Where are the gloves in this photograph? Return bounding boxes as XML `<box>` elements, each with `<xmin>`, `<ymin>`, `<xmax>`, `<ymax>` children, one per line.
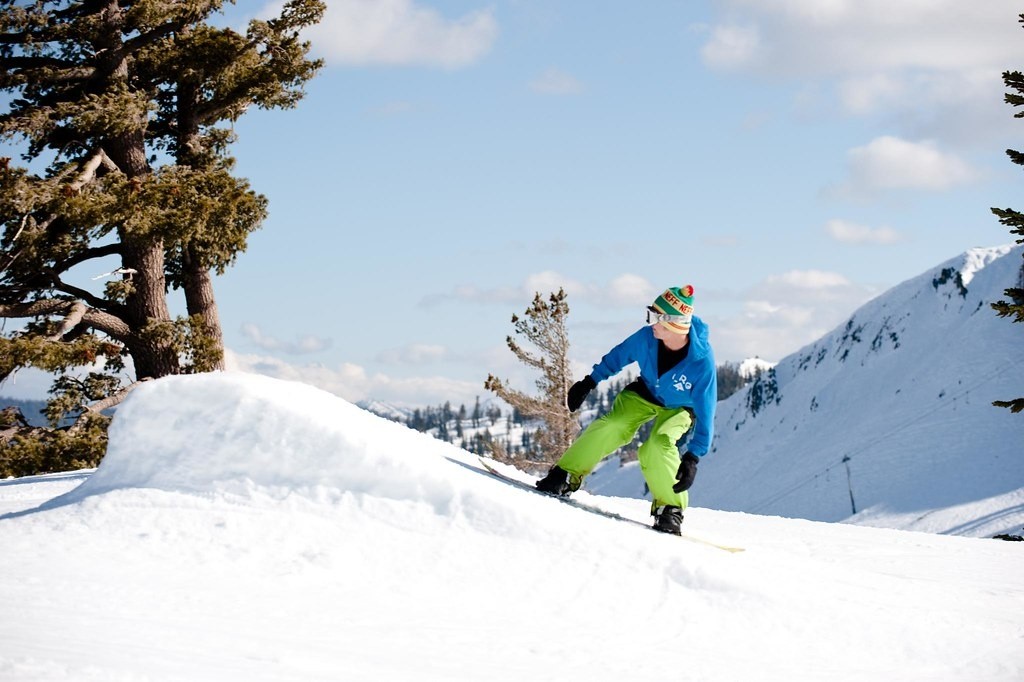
<box><xmin>568</xmin><ymin>375</ymin><xmax>596</xmax><ymax>412</ymax></box>
<box><xmin>672</xmin><ymin>452</ymin><xmax>699</xmax><ymax>493</ymax></box>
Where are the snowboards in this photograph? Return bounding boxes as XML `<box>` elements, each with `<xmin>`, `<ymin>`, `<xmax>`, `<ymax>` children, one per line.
<box><xmin>477</xmin><ymin>458</ymin><xmax>683</xmax><ymax>537</ymax></box>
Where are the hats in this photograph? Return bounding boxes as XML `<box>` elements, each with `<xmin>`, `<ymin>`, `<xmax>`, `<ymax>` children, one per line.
<box><xmin>653</xmin><ymin>285</ymin><xmax>695</xmax><ymax>334</ymax></box>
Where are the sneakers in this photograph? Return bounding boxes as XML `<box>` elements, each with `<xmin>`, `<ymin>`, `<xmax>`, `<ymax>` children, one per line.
<box><xmin>653</xmin><ymin>505</ymin><xmax>683</xmax><ymax>535</ymax></box>
<box><xmin>536</xmin><ymin>465</ymin><xmax>582</xmax><ymax>501</ymax></box>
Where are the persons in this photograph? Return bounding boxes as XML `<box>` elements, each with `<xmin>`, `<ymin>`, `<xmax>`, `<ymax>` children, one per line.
<box><xmin>535</xmin><ymin>285</ymin><xmax>718</xmax><ymax>535</ymax></box>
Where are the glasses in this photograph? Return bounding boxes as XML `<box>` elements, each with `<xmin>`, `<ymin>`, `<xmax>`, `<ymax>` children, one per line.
<box><xmin>645</xmin><ymin>306</ymin><xmax>692</xmax><ymax>326</ymax></box>
